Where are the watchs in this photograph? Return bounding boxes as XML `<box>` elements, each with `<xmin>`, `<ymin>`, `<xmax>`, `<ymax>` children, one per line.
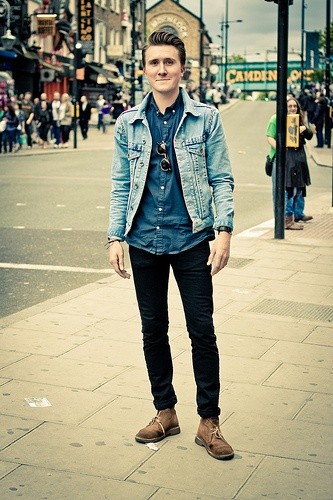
<box><xmin>211</xmin><ymin>222</ymin><xmax>233</xmax><ymax>239</ymax></box>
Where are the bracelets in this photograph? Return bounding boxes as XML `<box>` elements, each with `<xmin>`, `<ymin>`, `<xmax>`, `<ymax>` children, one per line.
<box><xmin>106</xmin><ymin>234</ymin><xmax>126</xmax><ymax>247</ymax></box>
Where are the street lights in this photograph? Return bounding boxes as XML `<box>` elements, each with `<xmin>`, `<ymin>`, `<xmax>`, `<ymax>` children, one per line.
<box><xmin>216</xmin><ymin>16</ymin><xmax>242</xmax><ymax>82</ymax></box>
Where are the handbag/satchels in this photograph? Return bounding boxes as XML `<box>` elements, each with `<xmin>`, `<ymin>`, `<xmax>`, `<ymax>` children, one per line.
<box><xmin>264</xmin><ymin>155</ymin><xmax>272</xmax><ymax>177</ymax></box>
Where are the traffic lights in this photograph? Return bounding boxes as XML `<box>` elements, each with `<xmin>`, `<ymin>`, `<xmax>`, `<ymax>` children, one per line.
<box><xmin>77</xmin><ymin>49</ymin><xmax>87</xmax><ymax>69</ymax></box>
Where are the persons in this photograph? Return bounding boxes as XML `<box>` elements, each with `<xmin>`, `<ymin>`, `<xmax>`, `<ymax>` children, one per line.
<box><xmin>295</xmin><ymin>104</ymin><xmax>313</xmax><ymax>222</ymax></box>
<box><xmin>1</xmin><ymin>86</ymin><xmax>333</xmax><ymax>152</ymax></box>
<box><xmin>105</xmin><ymin>32</ymin><xmax>234</xmax><ymax>460</ymax></box>
<box><xmin>264</xmin><ymin>99</ymin><xmax>304</xmax><ymax>234</ymax></box>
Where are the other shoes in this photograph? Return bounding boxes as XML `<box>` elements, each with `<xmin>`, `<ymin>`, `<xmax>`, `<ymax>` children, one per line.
<box><xmin>81</xmin><ymin>137</ymin><xmax>88</xmax><ymax>142</ymax></box>
<box><xmin>97</xmin><ymin>129</ymin><xmax>100</xmax><ymax>132</ymax></box>
<box><xmin>53</xmin><ymin>144</ymin><xmax>60</xmax><ymax>149</ymax></box>
<box><xmin>42</xmin><ymin>142</ymin><xmax>48</xmax><ymax>150</ymax></box>
<box><xmin>9</xmin><ymin>146</ymin><xmax>12</xmax><ymax>152</ymax></box>
<box><xmin>3</xmin><ymin>148</ymin><xmax>8</xmax><ymax>154</ymax></box>
<box><xmin>103</xmin><ymin>131</ymin><xmax>106</xmax><ymax>134</ymax></box>
<box><xmin>314</xmin><ymin>145</ymin><xmax>324</xmax><ymax>148</ymax></box>
<box><xmin>26</xmin><ymin>145</ymin><xmax>32</xmax><ymax>150</ymax></box>
<box><xmin>61</xmin><ymin>143</ymin><xmax>69</xmax><ymax>148</ymax></box>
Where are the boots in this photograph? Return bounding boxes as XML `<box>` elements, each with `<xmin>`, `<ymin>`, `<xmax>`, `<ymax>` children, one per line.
<box><xmin>284</xmin><ymin>217</ymin><xmax>304</xmax><ymax>230</ymax></box>
<box><xmin>194</xmin><ymin>416</ymin><xmax>235</xmax><ymax>461</ymax></box>
<box><xmin>135</xmin><ymin>410</ymin><xmax>182</xmax><ymax>443</ymax></box>
<box><xmin>294</xmin><ymin>215</ymin><xmax>313</xmax><ymax>222</ymax></box>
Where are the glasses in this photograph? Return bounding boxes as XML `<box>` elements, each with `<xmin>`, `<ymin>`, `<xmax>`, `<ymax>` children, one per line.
<box><xmin>156</xmin><ymin>143</ymin><xmax>171</xmax><ymax>172</ymax></box>
